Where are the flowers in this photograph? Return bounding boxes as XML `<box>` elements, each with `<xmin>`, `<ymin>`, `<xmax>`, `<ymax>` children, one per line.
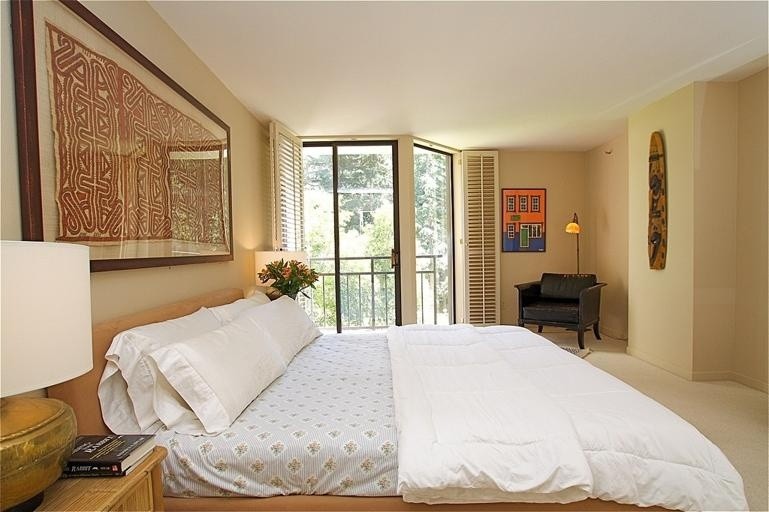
<box><xmin>257</xmin><ymin>257</ymin><xmax>323</xmax><ymax>301</ymax></box>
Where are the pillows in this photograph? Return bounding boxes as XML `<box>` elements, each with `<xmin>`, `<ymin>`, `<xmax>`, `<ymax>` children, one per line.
<box><xmin>249</xmin><ymin>295</ymin><xmax>323</xmax><ymax>367</ymax></box>
<box><xmin>97</xmin><ymin>305</ymin><xmax>215</xmax><ymax>428</ymax></box>
<box><xmin>209</xmin><ymin>288</ymin><xmax>270</xmax><ymax>323</ymax></box>
<box><xmin>148</xmin><ymin>318</ymin><xmax>288</xmax><ymax>436</ymax></box>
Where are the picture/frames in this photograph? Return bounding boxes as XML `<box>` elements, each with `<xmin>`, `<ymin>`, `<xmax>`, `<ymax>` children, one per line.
<box><xmin>501</xmin><ymin>187</ymin><xmax>548</xmax><ymax>252</ymax></box>
<box><xmin>11</xmin><ymin>0</ymin><xmax>237</xmax><ymax>272</ymax></box>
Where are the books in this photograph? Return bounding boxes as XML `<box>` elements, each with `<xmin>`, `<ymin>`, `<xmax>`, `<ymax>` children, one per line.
<box><xmin>58</xmin><ymin>446</ymin><xmax>155</xmax><ymax>477</ymax></box>
<box><xmin>58</xmin><ymin>433</ymin><xmax>157</xmax><ymax>472</ymax></box>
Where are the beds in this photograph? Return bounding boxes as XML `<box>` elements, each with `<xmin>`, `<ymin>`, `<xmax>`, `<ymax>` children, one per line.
<box><xmin>48</xmin><ymin>289</ymin><xmax>749</xmax><ymax>511</ymax></box>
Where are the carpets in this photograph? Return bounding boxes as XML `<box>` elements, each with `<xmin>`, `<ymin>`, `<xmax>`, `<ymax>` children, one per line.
<box><xmin>558</xmin><ymin>344</ymin><xmax>591</xmax><ymax>361</ymax></box>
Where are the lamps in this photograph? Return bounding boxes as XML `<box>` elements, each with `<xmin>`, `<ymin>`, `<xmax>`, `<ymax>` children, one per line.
<box><xmin>567</xmin><ymin>213</ymin><xmax>585</xmax><ymax>274</ymax></box>
<box><xmin>253</xmin><ymin>248</ymin><xmax>307</xmax><ymax>299</ymax></box>
<box><xmin>0</xmin><ymin>239</ymin><xmax>97</xmax><ymax>512</ymax></box>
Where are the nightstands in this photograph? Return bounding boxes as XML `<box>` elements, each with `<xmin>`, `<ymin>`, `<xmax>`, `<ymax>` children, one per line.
<box><xmin>40</xmin><ymin>446</ymin><xmax>170</xmax><ymax>512</ymax></box>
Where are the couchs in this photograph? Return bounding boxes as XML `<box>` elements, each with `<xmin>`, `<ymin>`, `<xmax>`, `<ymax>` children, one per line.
<box><xmin>507</xmin><ymin>274</ymin><xmax>605</xmax><ymax>349</ymax></box>
